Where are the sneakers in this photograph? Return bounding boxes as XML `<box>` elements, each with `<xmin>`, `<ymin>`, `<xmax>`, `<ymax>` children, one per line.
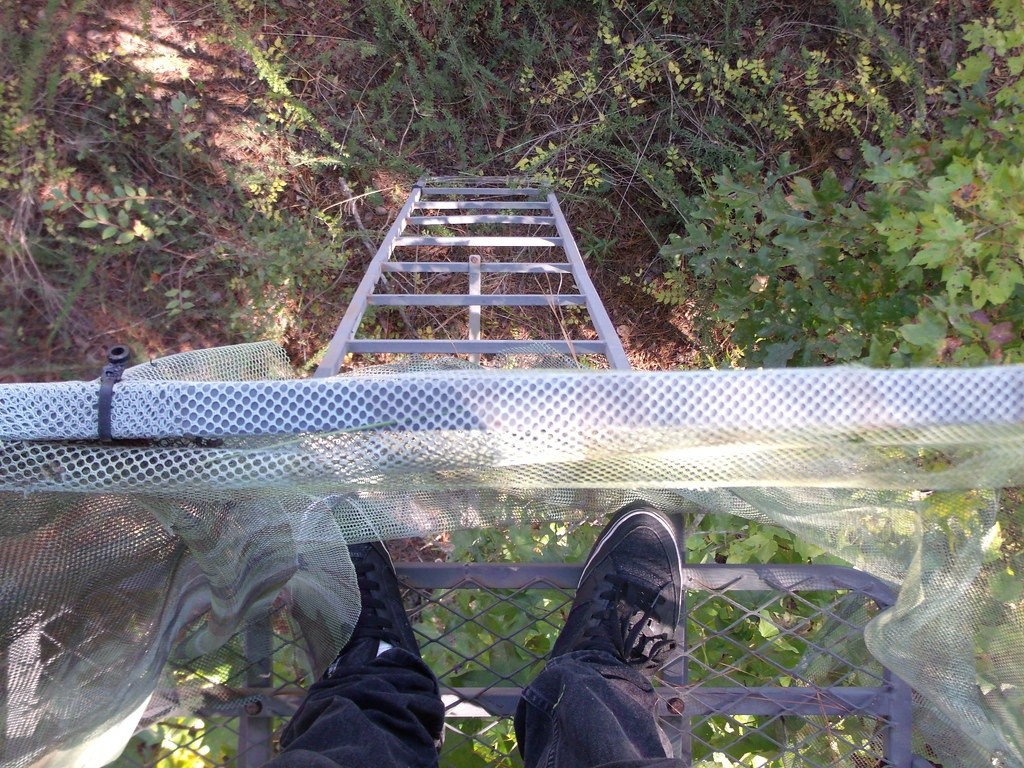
<box><xmin>551</xmin><ymin>500</ymin><xmax>685</xmax><ymax>689</ymax></box>
<box><xmin>293</xmin><ymin>496</ymin><xmax>422</xmax><ymax>684</ymax></box>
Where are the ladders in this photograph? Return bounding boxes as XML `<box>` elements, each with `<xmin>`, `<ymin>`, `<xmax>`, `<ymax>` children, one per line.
<box><xmin>310</xmin><ymin>173</ymin><xmax>636</xmax><ymax>380</ymax></box>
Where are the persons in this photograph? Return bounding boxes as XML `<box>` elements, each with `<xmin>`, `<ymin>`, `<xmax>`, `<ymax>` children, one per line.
<box><xmin>259</xmin><ymin>493</ymin><xmax>694</xmax><ymax>768</ymax></box>
